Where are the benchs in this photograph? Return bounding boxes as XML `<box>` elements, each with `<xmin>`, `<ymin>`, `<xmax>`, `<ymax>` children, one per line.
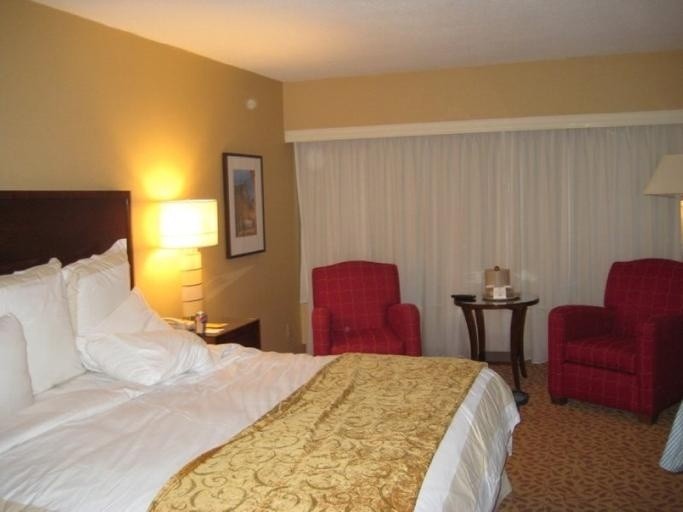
<box><xmin>545</xmin><ymin>256</ymin><xmax>682</xmax><ymax>425</ymax></box>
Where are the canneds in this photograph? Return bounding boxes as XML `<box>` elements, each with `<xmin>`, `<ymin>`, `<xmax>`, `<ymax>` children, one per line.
<box><xmin>196</xmin><ymin>312</ymin><xmax>207</xmax><ymax>334</ymax></box>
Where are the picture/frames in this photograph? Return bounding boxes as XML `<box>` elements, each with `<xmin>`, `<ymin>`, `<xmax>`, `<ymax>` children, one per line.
<box><xmin>220</xmin><ymin>151</ymin><xmax>266</xmax><ymax>258</ymax></box>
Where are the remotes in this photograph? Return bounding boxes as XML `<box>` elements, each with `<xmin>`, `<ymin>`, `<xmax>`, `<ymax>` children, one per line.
<box><xmin>451</xmin><ymin>294</ymin><xmax>476</xmax><ymax>299</ymax></box>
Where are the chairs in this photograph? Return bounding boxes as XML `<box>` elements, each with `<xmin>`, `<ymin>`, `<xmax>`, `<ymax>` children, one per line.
<box><xmin>309</xmin><ymin>260</ymin><xmax>423</xmax><ymax>356</ymax></box>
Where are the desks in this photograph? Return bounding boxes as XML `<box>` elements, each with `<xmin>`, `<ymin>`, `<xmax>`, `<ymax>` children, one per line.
<box><xmin>450</xmin><ymin>291</ymin><xmax>539</xmax><ymax>396</ymax></box>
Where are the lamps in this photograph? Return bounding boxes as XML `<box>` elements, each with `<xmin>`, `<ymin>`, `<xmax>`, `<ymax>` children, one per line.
<box><xmin>642</xmin><ymin>155</ymin><xmax>682</xmax><ymax>239</ymax></box>
<box><xmin>158</xmin><ymin>197</ymin><xmax>219</xmax><ymax>321</ymax></box>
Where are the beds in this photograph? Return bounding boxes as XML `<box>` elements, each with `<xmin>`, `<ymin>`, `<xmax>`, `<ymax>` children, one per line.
<box><xmin>0</xmin><ymin>190</ymin><xmax>521</xmax><ymax>512</ymax></box>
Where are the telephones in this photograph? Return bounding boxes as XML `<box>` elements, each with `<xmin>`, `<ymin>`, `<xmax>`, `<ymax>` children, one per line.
<box><xmin>163</xmin><ymin>317</ymin><xmax>195</xmax><ymax>330</ymax></box>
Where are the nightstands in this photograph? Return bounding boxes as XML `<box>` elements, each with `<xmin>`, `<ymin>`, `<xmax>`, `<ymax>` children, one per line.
<box><xmin>179</xmin><ymin>316</ymin><xmax>261</xmax><ymax>350</ymax></box>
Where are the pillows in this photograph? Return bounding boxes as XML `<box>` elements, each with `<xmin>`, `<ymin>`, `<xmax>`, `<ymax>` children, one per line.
<box><xmin>0</xmin><ymin>238</ymin><xmax>210</xmax><ymax>424</ymax></box>
